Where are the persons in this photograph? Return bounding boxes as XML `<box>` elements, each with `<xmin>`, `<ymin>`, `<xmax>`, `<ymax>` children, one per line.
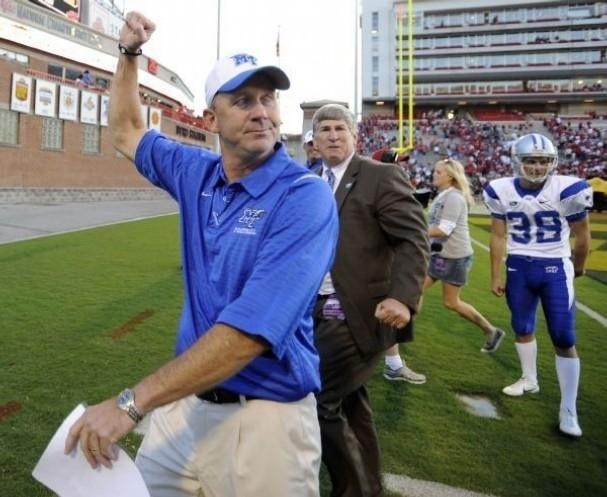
<box><xmin>299</xmin><ymin>128</ymin><xmax>324</xmax><ymax>170</ymax></box>
<box><xmin>76</xmin><ymin>72</ymin><xmax>84</xmax><ymax>83</ymax></box>
<box><xmin>415</xmin><ymin>157</ymin><xmax>507</xmax><ymax>354</ymax></box>
<box><xmin>352</xmin><ymin>80</ymin><xmax>607</xmax><ymax>213</ymax></box>
<box><xmin>307</xmin><ymin>103</ymin><xmax>430</xmax><ymax>496</ymax></box>
<box><xmin>483</xmin><ymin>134</ymin><xmax>594</xmax><ymax>438</ymax></box>
<box><xmin>63</xmin><ymin>10</ymin><xmax>342</xmax><ymax>497</ymax></box>
<box><xmin>175</xmin><ymin>104</ymin><xmax>188</xmax><ymax>115</ymax></box>
<box><xmin>373</xmin><ymin>146</ymin><xmax>428</xmax><ymax>384</ymax></box>
<box><xmin>84</xmin><ymin>70</ymin><xmax>94</xmax><ymax>86</ymax></box>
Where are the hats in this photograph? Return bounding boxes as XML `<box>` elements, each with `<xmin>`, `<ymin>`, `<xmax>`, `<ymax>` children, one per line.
<box><xmin>206</xmin><ymin>55</ymin><xmax>289</xmax><ymax>106</ymax></box>
<box><xmin>304</xmin><ymin>130</ymin><xmax>313</xmax><ymax>143</ymax></box>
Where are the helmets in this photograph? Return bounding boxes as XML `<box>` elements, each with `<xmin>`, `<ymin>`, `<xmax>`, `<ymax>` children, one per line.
<box><xmin>511</xmin><ymin>134</ymin><xmax>557</xmax><ymax>182</ymax></box>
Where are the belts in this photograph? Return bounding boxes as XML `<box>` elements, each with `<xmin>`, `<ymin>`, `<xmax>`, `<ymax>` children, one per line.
<box><xmin>316</xmin><ymin>293</ymin><xmax>340</xmax><ymax>303</ymax></box>
<box><xmin>197</xmin><ymin>387</ymin><xmax>263</xmax><ymax>404</ymax></box>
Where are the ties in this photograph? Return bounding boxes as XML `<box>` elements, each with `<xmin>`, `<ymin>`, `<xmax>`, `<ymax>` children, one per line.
<box><xmin>325</xmin><ymin>169</ymin><xmax>336</xmax><ymax>190</ymax></box>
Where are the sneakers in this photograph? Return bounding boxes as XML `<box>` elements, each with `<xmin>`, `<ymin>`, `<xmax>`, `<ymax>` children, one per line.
<box><xmin>558</xmin><ymin>407</ymin><xmax>582</xmax><ymax>436</ymax></box>
<box><xmin>383</xmin><ymin>364</ymin><xmax>426</xmax><ymax>384</ymax></box>
<box><xmin>502</xmin><ymin>377</ymin><xmax>540</xmax><ymax>396</ymax></box>
<box><xmin>480</xmin><ymin>328</ymin><xmax>504</xmax><ymax>353</ymax></box>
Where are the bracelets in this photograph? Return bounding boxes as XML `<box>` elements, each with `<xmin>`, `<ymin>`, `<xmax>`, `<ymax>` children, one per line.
<box><xmin>575</xmin><ymin>269</ymin><xmax>585</xmax><ymax>278</ymax></box>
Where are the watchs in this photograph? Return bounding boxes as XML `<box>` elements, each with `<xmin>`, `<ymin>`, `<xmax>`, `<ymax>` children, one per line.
<box><xmin>115</xmin><ymin>388</ymin><xmax>143</xmax><ymax>423</ymax></box>
<box><xmin>117</xmin><ymin>44</ymin><xmax>141</xmax><ymax>57</ymax></box>
